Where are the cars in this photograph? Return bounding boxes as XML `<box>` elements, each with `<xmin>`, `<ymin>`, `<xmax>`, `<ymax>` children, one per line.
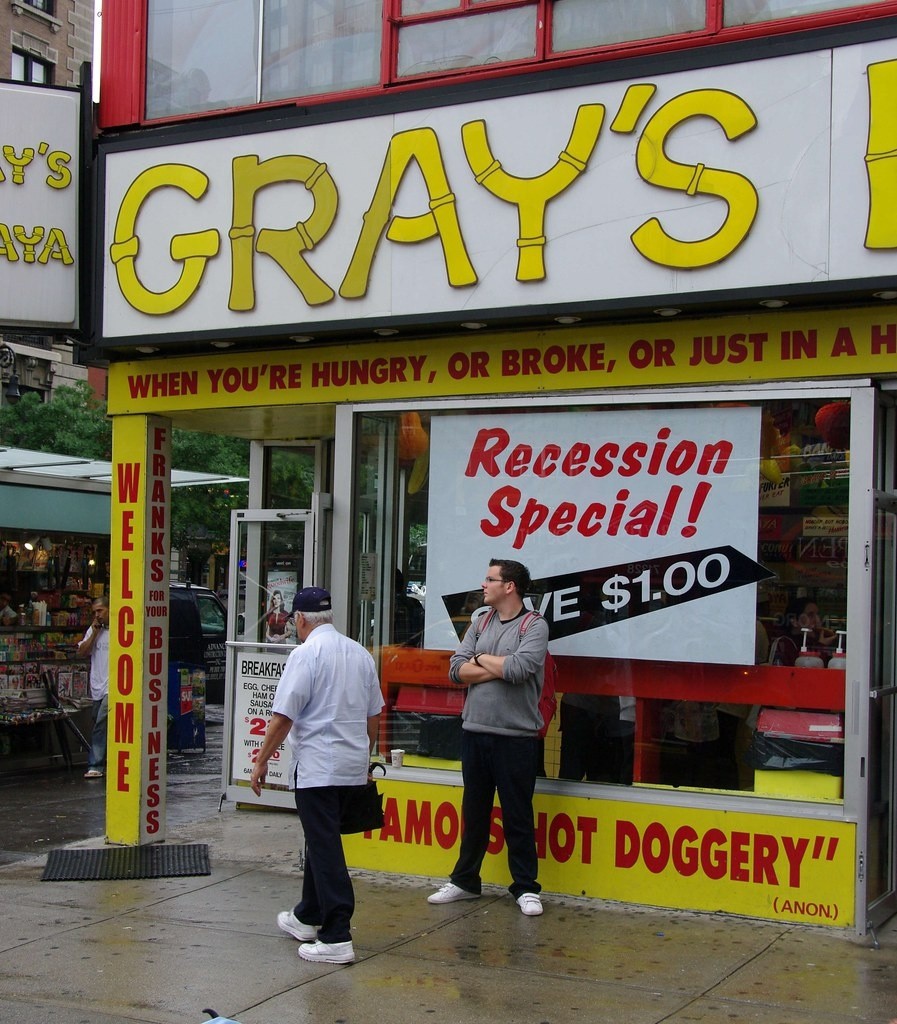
<box><xmin>239</xmin><ymin>580</ymin><xmax>246</xmax><ymax>596</ymax></box>
<box><xmin>399</xmin><ymin>616</ymin><xmax>471</xmax><ymax>652</ymax></box>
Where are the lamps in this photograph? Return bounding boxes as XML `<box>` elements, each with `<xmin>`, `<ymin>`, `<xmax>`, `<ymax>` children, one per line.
<box><xmin>24</xmin><ymin>535</ymin><xmax>40</xmax><ymax>551</ymax></box>
<box><xmin>41</xmin><ymin>537</ymin><xmax>53</xmax><ymax>551</ymax></box>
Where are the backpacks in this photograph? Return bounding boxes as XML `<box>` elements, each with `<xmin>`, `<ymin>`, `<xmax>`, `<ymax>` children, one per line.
<box><xmin>476</xmin><ymin>608</ymin><xmax>557</xmax><ymax>738</ymax></box>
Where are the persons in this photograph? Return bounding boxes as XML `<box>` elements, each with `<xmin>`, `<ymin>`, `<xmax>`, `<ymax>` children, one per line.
<box><xmin>264</xmin><ymin>590</ymin><xmax>290</xmax><ymax>653</ymax></box>
<box><xmin>77</xmin><ymin>595</ymin><xmax>109</xmax><ymax>777</ymax></box>
<box><xmin>559</xmin><ymin>692</ymin><xmax>622</xmax><ymax>782</ymax></box>
<box><xmin>691</xmin><ymin>597</ymin><xmax>837</xmax><ymax>791</ymax></box>
<box><xmin>427</xmin><ymin>558</ymin><xmax>550</xmax><ymax>916</ymax></box>
<box><xmin>383</xmin><ymin>568</ymin><xmax>424</xmax><ymax>648</ymax></box>
<box><xmin>252</xmin><ymin>588</ymin><xmax>385</xmax><ymax>965</ymax></box>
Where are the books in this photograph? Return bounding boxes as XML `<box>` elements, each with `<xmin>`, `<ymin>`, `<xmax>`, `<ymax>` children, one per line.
<box><xmin>0</xmin><ymin>540</ymin><xmax>98</xmax><ymax>709</ymax></box>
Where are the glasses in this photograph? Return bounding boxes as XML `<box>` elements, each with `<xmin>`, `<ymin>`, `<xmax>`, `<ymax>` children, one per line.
<box><xmin>485</xmin><ymin>576</ymin><xmax>507</xmax><ymax>583</ymax></box>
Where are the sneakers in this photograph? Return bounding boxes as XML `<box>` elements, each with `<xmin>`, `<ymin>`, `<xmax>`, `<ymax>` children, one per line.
<box><xmin>298</xmin><ymin>940</ymin><xmax>355</xmax><ymax>964</ymax></box>
<box><xmin>277</xmin><ymin>908</ymin><xmax>317</xmax><ymax>941</ymax></box>
<box><xmin>515</xmin><ymin>893</ymin><xmax>543</xmax><ymax>915</ymax></box>
<box><xmin>427</xmin><ymin>882</ymin><xmax>481</xmax><ymax>904</ymax></box>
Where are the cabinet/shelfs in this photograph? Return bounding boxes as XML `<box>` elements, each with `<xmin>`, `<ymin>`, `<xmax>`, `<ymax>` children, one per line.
<box><xmin>0</xmin><ymin>628</ymin><xmax>92</xmax><ymax>724</ymax></box>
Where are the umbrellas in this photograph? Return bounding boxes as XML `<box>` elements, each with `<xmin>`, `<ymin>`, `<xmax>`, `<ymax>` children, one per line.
<box><xmin>339</xmin><ymin>761</ymin><xmax>386</xmax><ymax>834</ymax></box>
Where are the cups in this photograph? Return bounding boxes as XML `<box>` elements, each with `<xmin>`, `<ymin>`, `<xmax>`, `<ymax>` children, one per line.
<box><xmin>391</xmin><ymin>750</ymin><xmax>405</xmax><ymax>768</ymax></box>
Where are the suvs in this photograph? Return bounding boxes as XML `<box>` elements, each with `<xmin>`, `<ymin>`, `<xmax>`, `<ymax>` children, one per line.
<box><xmin>169</xmin><ymin>580</ymin><xmax>227</xmax><ymax>703</ymax></box>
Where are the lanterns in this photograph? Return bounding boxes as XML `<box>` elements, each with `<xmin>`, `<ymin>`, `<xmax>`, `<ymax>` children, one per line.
<box><xmin>815</xmin><ymin>404</ymin><xmax>851</xmax><ymax>450</ymax></box>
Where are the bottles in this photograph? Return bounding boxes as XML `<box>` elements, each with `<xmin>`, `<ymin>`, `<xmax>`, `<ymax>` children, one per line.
<box><xmin>827</xmin><ymin>652</ymin><xmax>846</xmax><ymax>670</ymax></box>
<box><xmin>17</xmin><ymin>600</ymin><xmax>39</xmax><ymax>626</ymax></box>
<box><xmin>794</xmin><ymin>651</ymin><xmax>824</xmax><ymax>668</ymax></box>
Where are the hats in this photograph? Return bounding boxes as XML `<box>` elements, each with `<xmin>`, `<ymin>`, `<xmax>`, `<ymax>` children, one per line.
<box><xmin>284</xmin><ymin>586</ymin><xmax>331</xmax><ymax>619</ymax></box>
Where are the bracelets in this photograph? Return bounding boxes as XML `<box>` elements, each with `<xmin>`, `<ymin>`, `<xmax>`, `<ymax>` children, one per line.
<box><xmin>473</xmin><ymin>651</ymin><xmax>487</xmax><ymax>668</ymax></box>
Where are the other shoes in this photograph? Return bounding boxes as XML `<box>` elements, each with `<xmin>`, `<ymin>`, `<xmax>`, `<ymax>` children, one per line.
<box><xmin>85</xmin><ymin>769</ymin><xmax>103</xmax><ymax>778</ymax></box>
